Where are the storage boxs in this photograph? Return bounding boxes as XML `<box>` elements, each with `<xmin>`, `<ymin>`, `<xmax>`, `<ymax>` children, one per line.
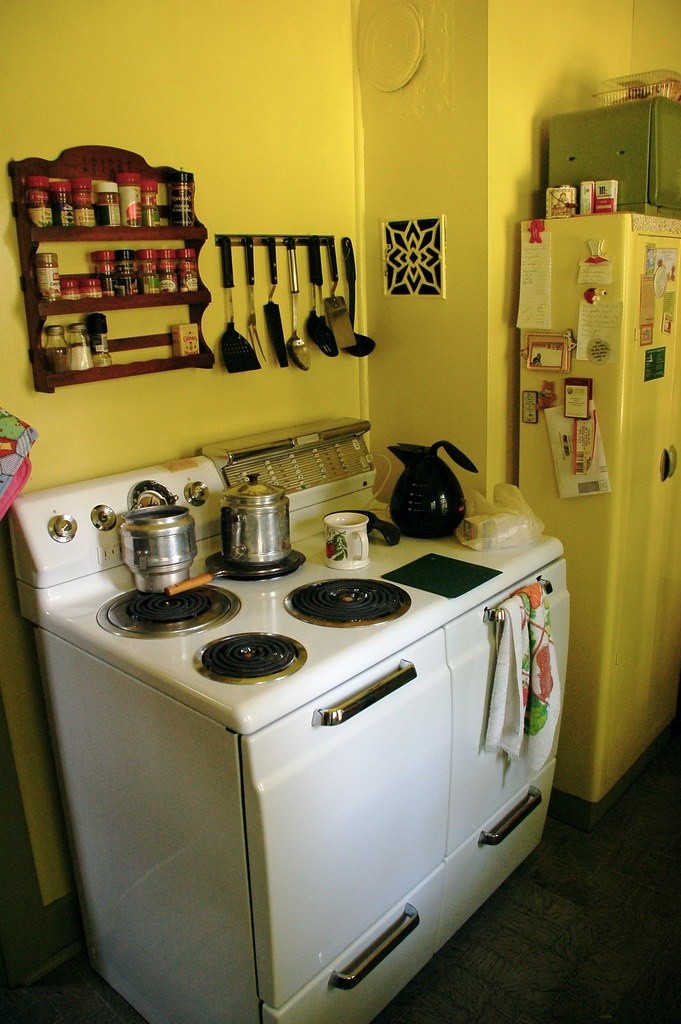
<box><xmin>596</xmin><ymin>180</ymin><xmax>618</xmax><ymax>213</ymax></box>
<box><xmin>463</xmin><ymin>515</ymin><xmax>493</xmax><ymax>549</ymax></box>
<box><xmin>579</xmin><ymin>181</ymin><xmax>595</xmax><ymax>213</ymax></box>
<box><xmin>545</xmin><ymin>187</ymin><xmax>577</xmax><ymax>218</ymax></box>
<box><xmin>547</xmin><ymin>96</ymin><xmax>681</xmax><ymax>219</ymax></box>
<box><xmin>172</xmin><ymin>323</ymin><xmax>199</xmax><ymax>357</ymax></box>
<box><xmin>592</xmin><ymin>69</ymin><xmax>681</xmax><ymax>108</ymax></box>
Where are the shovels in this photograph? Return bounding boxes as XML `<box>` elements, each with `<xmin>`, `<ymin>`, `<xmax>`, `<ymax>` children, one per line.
<box><xmin>325</xmin><ymin>237</ymin><xmax>356</xmax><ymax>348</ymax></box>
<box><xmin>265</xmin><ymin>237</ymin><xmax>287</xmax><ymax>369</ymax></box>
<box><xmin>219</xmin><ymin>234</ymin><xmax>260</xmax><ymax>372</ymax></box>
<box><xmin>307</xmin><ymin>235</ymin><xmax>338</xmax><ymax>360</ymax></box>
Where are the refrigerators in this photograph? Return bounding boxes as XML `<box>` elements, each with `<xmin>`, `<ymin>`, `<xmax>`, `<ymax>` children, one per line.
<box><xmin>520</xmin><ymin>213</ymin><xmax>681</xmax><ymax>802</ymax></box>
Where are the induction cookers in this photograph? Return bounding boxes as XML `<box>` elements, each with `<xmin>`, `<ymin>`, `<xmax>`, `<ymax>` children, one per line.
<box><xmin>202</xmin><ymin>417</ymin><xmax>563</xmax><ymax>627</ymax></box>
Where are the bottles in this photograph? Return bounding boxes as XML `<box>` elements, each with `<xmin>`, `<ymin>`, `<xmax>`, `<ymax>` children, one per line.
<box><xmin>44</xmin><ymin>326</ymin><xmax>70</xmax><ymax>372</ymax></box>
<box><xmin>168</xmin><ymin>172</ymin><xmax>195</xmax><ymax>227</ymax></box>
<box><xmin>35</xmin><ymin>253</ymin><xmax>61</xmax><ymax>302</ymax></box>
<box><xmin>136</xmin><ymin>249</ymin><xmax>160</xmax><ymax>294</ymax></box>
<box><xmin>117</xmin><ymin>173</ymin><xmax>142</xmax><ymax>227</ymax></box>
<box><xmin>71</xmin><ymin>179</ymin><xmax>96</xmax><ymax>227</ymax></box>
<box><xmin>27</xmin><ymin>176</ymin><xmax>53</xmax><ymax>228</ymax></box>
<box><xmin>67</xmin><ymin>323</ymin><xmax>94</xmax><ymax>371</ymax></box>
<box><xmin>60</xmin><ymin>275</ymin><xmax>81</xmax><ymax>300</ymax></box>
<box><xmin>93</xmin><ymin>183</ymin><xmax>121</xmax><ymax>226</ymax></box>
<box><xmin>81</xmin><ymin>273</ymin><xmax>103</xmax><ymax>299</ymax></box>
<box><xmin>140</xmin><ymin>181</ymin><xmax>160</xmax><ymax>227</ymax></box>
<box><xmin>49</xmin><ymin>182</ymin><xmax>74</xmax><ymax>227</ymax></box>
<box><xmin>158</xmin><ymin>250</ymin><xmax>178</xmax><ymax>294</ymax></box>
<box><xmin>115</xmin><ymin>250</ymin><xmax>137</xmax><ymax>296</ymax></box>
<box><xmin>91</xmin><ymin>251</ymin><xmax>118</xmax><ymax>296</ymax></box>
<box><xmin>177</xmin><ymin>249</ymin><xmax>198</xmax><ymax>292</ymax></box>
<box><xmin>85</xmin><ymin>313</ymin><xmax>112</xmax><ymax>368</ymax></box>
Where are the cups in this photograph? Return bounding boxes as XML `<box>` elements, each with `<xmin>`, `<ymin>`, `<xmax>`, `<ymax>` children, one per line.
<box><xmin>323</xmin><ymin>512</ymin><xmax>370</xmax><ymax>570</ymax></box>
<box><xmin>220</xmin><ymin>472</ymin><xmax>291</xmax><ymax>567</ymax></box>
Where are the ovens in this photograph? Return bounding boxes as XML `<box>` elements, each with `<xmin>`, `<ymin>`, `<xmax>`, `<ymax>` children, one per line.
<box><xmin>33</xmin><ymin>559</ymin><xmax>569</xmax><ymax>1024</ymax></box>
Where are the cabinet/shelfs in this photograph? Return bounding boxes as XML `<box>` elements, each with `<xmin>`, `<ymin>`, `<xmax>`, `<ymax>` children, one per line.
<box><xmin>6</xmin><ymin>145</ymin><xmax>214</xmax><ymax>394</ymax></box>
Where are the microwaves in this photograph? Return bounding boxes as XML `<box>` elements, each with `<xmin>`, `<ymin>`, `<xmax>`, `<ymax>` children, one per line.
<box><xmin>549</xmin><ymin>96</ymin><xmax>681</xmax><ymax>219</ymax></box>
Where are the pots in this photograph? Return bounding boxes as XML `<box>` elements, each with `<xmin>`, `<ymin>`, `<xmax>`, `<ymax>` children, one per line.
<box><xmin>120</xmin><ymin>505</ymin><xmax>198</xmax><ymax>593</ymax></box>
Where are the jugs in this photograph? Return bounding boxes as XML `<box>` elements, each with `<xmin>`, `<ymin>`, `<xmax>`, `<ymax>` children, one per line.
<box><xmin>387</xmin><ymin>441</ymin><xmax>479</xmax><ymax>540</ymax></box>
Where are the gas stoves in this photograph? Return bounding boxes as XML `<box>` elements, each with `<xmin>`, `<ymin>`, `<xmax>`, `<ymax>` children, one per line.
<box><xmin>10</xmin><ymin>454</ymin><xmax>442</xmax><ymax>737</ymax></box>
